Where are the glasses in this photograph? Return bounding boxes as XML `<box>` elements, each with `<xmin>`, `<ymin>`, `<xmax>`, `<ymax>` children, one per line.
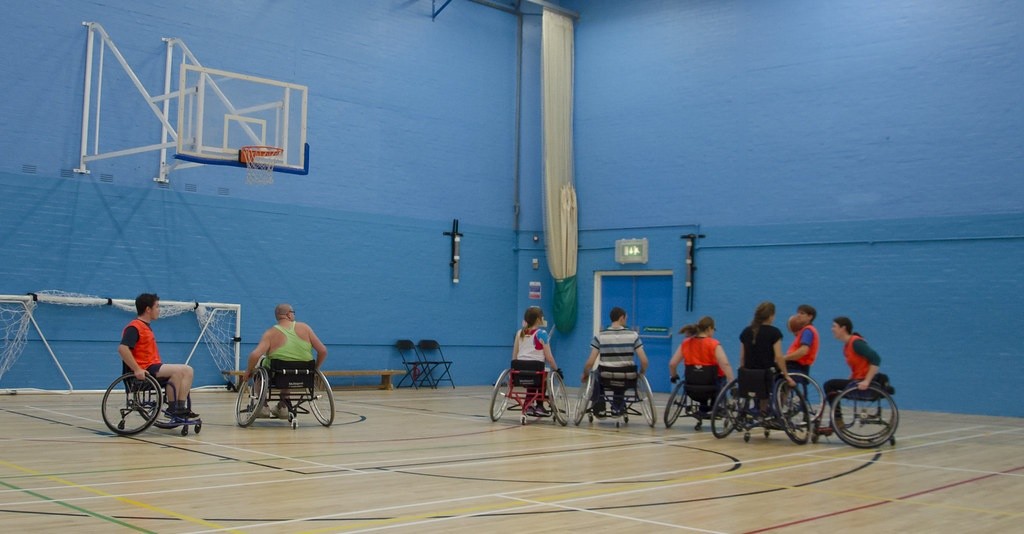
<box><xmin>711</xmin><ymin>327</ymin><xmax>716</xmax><ymax>331</ymax></box>
<box><xmin>289</xmin><ymin>310</ymin><xmax>296</xmax><ymax>315</ymax></box>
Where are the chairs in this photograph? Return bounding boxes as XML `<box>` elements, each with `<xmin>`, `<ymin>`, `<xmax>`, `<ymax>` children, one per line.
<box><xmin>396</xmin><ymin>339</ymin><xmax>456</xmax><ymax>389</ymax></box>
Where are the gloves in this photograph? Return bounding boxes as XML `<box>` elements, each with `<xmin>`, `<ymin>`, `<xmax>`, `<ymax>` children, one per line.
<box><xmin>730</xmin><ymin>385</ymin><xmax>738</xmax><ymax>396</ymax></box>
<box><xmin>671</xmin><ymin>375</ymin><xmax>680</xmax><ymax>384</ymax></box>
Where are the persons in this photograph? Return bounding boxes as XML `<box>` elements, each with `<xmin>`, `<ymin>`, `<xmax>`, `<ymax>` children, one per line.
<box><xmin>805</xmin><ymin>316</ymin><xmax>881</xmax><ymax>434</ymax></box>
<box><xmin>783</xmin><ymin>304</ymin><xmax>819</xmax><ymax>382</ymax></box>
<box><xmin>668</xmin><ymin>315</ymin><xmax>734</xmax><ymax>403</ymax></box>
<box><xmin>738</xmin><ymin>301</ymin><xmax>796</xmax><ymax>417</ymax></box>
<box><xmin>241</xmin><ymin>303</ymin><xmax>326</xmax><ymax>415</ymax></box>
<box><xmin>119</xmin><ymin>293</ymin><xmax>201</xmax><ymax>421</ymax></box>
<box><xmin>579</xmin><ymin>306</ymin><xmax>647</xmax><ymax>419</ymax></box>
<box><xmin>512</xmin><ymin>305</ymin><xmax>564</xmax><ymax>417</ymax></box>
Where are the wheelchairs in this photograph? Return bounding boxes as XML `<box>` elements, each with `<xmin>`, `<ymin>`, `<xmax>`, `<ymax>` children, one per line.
<box><xmin>101</xmin><ymin>358</ymin><xmax>203</xmax><ymax>436</ymax></box>
<box><xmin>575</xmin><ymin>364</ymin><xmax>657</xmax><ymax>428</ymax></box>
<box><xmin>664</xmin><ymin>364</ymin><xmax>727</xmax><ymax>427</ymax></box>
<box><xmin>490</xmin><ymin>359</ymin><xmax>571</xmax><ymax>427</ymax></box>
<box><xmin>812</xmin><ymin>372</ymin><xmax>899</xmax><ymax>448</ymax></box>
<box><xmin>235</xmin><ymin>358</ymin><xmax>335</xmax><ymax>430</ymax></box>
<box><xmin>711</xmin><ymin>366</ymin><xmax>825</xmax><ymax>445</ymax></box>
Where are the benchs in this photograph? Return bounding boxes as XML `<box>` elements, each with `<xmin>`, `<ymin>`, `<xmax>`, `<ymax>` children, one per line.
<box><xmin>221</xmin><ymin>369</ymin><xmax>407</xmax><ymax>391</ymax></box>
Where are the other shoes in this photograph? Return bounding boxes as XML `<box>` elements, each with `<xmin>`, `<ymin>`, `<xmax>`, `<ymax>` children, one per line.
<box><xmin>165</xmin><ymin>407</ymin><xmax>200</xmax><ymax>421</ymax></box>
<box><xmin>272</xmin><ymin>403</ymin><xmax>288</xmax><ymax>419</ymax></box>
<box><xmin>255</xmin><ymin>405</ymin><xmax>270</xmax><ymax>417</ymax></box>
<box><xmin>526</xmin><ymin>406</ymin><xmax>550</xmax><ymax>416</ymax></box>
<box><xmin>814</xmin><ymin>418</ymin><xmax>846</xmax><ymax>433</ymax></box>
<box><xmin>699</xmin><ymin>405</ymin><xmax>724</xmax><ymax>414</ymax></box>
<box><xmin>611</xmin><ymin>404</ymin><xmax>627</xmax><ymax>417</ymax></box>
<box><xmin>592</xmin><ymin>404</ymin><xmax>607</xmax><ymax>420</ymax></box>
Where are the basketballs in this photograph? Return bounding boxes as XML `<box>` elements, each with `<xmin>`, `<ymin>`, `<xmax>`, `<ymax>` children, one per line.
<box><xmin>786</xmin><ymin>315</ymin><xmax>804</xmax><ymax>333</ymax></box>
